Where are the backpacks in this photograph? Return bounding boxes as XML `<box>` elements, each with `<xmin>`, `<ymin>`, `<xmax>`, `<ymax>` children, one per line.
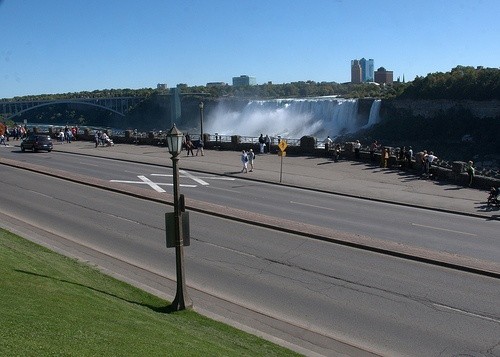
<box><xmin>245</xmin><ymin>156</ymin><xmax>248</xmax><ymax>162</ymax></box>
<box><xmin>252</xmin><ymin>152</ymin><xmax>255</xmax><ymax>159</ymax></box>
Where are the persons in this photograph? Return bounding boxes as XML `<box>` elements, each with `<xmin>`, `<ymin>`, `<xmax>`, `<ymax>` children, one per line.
<box><xmin>382</xmin><ymin>146</ymin><xmax>438</xmax><ymax>179</ymax></box>
<box><xmin>354</xmin><ymin>140</ymin><xmax>361</xmax><ymax>160</ymax></box>
<box><xmin>95</xmin><ymin>130</ymin><xmax>107</xmax><ymax>147</ymax></box>
<box><xmin>324</xmin><ymin>136</ymin><xmax>333</xmax><ymax>154</ymax></box>
<box><xmin>488</xmin><ymin>187</ymin><xmax>498</xmax><ymax>199</ymax></box>
<box><xmin>196</xmin><ymin>137</ymin><xmax>204</xmax><ymax>156</ymax></box>
<box><xmin>259</xmin><ymin>134</ymin><xmax>271</xmax><ymax>153</ymax></box>
<box><xmin>0</xmin><ymin>125</ymin><xmax>26</xmax><ymax>144</ymax></box>
<box><xmin>241</xmin><ymin>149</ymin><xmax>254</xmax><ymax>173</ymax></box>
<box><xmin>334</xmin><ymin>143</ymin><xmax>340</xmax><ymax>162</ymax></box>
<box><xmin>369</xmin><ymin>140</ymin><xmax>381</xmax><ymax>159</ymax></box>
<box><xmin>186</xmin><ymin>139</ymin><xmax>194</xmax><ymax>157</ymax></box>
<box><xmin>55</xmin><ymin>125</ymin><xmax>76</xmax><ymax>144</ymax></box>
<box><xmin>466</xmin><ymin>161</ymin><xmax>475</xmax><ymax>187</ymax></box>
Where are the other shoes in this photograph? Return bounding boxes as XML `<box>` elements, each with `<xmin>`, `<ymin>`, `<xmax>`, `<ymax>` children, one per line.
<box><xmin>249</xmin><ymin>169</ymin><xmax>253</xmax><ymax>172</ymax></box>
<box><xmin>241</xmin><ymin>170</ymin><xmax>247</xmax><ymax>173</ymax></box>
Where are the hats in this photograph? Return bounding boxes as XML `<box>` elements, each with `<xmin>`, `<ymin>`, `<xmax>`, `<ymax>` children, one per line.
<box><xmin>250</xmin><ymin>149</ymin><xmax>253</xmax><ymax>152</ymax></box>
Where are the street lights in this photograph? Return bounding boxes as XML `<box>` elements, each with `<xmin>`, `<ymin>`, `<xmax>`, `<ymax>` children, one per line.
<box><xmin>199</xmin><ymin>101</ymin><xmax>205</xmax><ymax>140</ymax></box>
<box><xmin>165</xmin><ymin>122</ymin><xmax>193</xmax><ymax>311</ymax></box>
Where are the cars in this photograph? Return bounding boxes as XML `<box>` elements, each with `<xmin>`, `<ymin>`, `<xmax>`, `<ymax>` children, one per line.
<box><xmin>21</xmin><ymin>134</ymin><xmax>52</xmax><ymax>153</ymax></box>
